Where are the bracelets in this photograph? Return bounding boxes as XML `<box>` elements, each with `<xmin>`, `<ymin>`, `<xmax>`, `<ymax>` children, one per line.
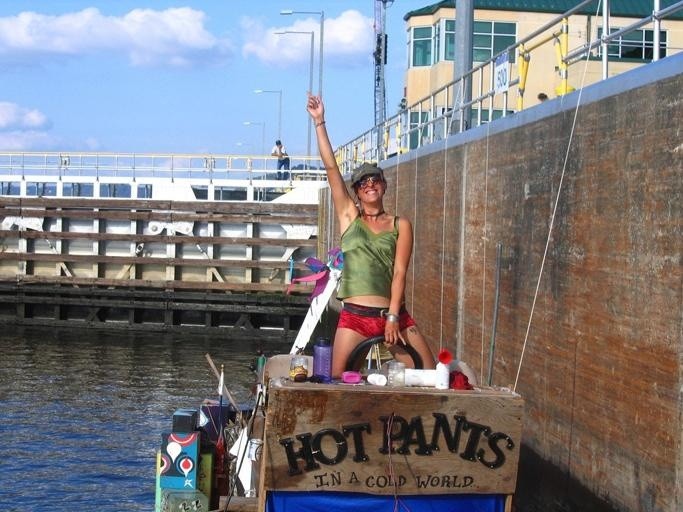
<box><xmin>386</xmin><ymin>313</ymin><xmax>398</xmax><ymax>322</ymax></box>
<box><xmin>314</xmin><ymin>120</ymin><xmax>326</xmax><ymax>128</ymax></box>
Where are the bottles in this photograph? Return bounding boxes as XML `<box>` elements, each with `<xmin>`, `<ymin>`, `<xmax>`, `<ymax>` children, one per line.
<box><xmin>313</xmin><ymin>338</ymin><xmax>332</xmax><ymax>383</ymax></box>
<box><xmin>368</xmin><ymin>373</ymin><xmax>388</xmax><ymax>385</ymax></box>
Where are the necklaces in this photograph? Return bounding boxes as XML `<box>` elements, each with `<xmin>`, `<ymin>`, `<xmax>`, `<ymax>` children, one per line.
<box><xmin>363</xmin><ymin>210</ymin><xmax>385</xmax><ymax>217</ymax></box>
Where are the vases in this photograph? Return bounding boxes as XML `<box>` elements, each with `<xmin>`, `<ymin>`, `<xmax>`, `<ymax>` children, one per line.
<box><xmin>436</xmin><ymin>361</ymin><xmax>450</xmax><ymax>390</ymax></box>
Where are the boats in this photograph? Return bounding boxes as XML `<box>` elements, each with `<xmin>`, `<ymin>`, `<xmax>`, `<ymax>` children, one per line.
<box><xmin>156</xmin><ymin>323</ymin><xmax>525</xmax><ymax>512</ymax></box>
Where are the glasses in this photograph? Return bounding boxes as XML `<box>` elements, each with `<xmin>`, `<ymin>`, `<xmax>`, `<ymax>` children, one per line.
<box><xmin>355</xmin><ymin>173</ymin><xmax>382</xmax><ymax>191</ymax></box>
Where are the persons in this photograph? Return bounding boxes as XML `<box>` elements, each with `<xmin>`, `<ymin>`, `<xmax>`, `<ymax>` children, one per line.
<box><xmin>270</xmin><ymin>140</ymin><xmax>289</xmax><ymax>180</ymax></box>
<box><xmin>304</xmin><ymin>90</ymin><xmax>435</xmax><ymax>380</ymax></box>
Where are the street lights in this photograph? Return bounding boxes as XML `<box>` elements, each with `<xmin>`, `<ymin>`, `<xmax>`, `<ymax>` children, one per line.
<box><xmin>253</xmin><ymin>88</ymin><xmax>283</xmax><ymax>152</ymax></box>
<box><xmin>243</xmin><ymin>120</ymin><xmax>266</xmax><ymax>155</ymax></box>
<box><xmin>273</xmin><ymin>9</ymin><xmax>326</xmax><ymax>171</ymax></box>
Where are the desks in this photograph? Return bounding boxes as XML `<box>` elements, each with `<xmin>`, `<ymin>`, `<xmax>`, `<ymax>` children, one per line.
<box><xmin>258</xmin><ymin>383</ymin><xmax>526</xmax><ymax>512</ymax></box>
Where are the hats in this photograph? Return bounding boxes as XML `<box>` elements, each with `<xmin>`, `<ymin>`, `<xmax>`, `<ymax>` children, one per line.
<box><xmin>350</xmin><ymin>163</ymin><xmax>384</xmax><ymax>189</ymax></box>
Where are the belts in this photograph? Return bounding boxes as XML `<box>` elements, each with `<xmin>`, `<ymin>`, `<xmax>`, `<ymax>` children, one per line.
<box><xmin>344</xmin><ymin>303</ymin><xmax>408</xmax><ymax>318</ymax></box>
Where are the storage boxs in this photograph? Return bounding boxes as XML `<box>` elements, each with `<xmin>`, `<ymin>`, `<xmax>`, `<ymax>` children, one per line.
<box><xmin>154</xmin><ymin>397</ymin><xmax>253</xmax><ymax>512</ymax></box>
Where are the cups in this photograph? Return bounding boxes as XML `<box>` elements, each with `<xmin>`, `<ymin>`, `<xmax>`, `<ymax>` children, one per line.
<box><xmin>289</xmin><ymin>357</ymin><xmax>308</xmax><ymax>382</ymax></box>
<box><xmin>388</xmin><ymin>361</ymin><xmax>405</xmax><ymax>385</ymax></box>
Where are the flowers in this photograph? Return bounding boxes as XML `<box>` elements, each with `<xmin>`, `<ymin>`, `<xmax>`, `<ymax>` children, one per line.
<box><xmin>438</xmin><ymin>348</ymin><xmax>452</xmax><ymax>364</ymax></box>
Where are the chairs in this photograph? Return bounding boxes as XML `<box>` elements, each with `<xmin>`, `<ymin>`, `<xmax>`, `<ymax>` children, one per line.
<box><xmin>261</xmin><ymin>354</ymin><xmax>318</xmax><ymax>387</ymax></box>
